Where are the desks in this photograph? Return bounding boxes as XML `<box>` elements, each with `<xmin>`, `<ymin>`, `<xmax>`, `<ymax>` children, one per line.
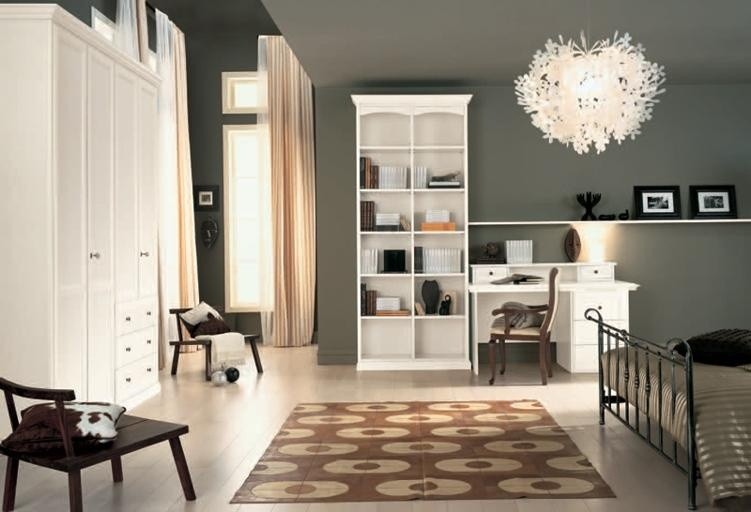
<box><xmin>469</xmin><ymin>259</ymin><xmax>639</xmax><ymax>373</ymax></box>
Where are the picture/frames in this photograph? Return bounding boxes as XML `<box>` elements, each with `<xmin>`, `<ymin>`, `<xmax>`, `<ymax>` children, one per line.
<box><xmin>690</xmin><ymin>184</ymin><xmax>738</xmax><ymax>220</ymax></box>
<box><xmin>193</xmin><ymin>184</ymin><xmax>219</xmax><ymax>211</ymax></box>
<box><xmin>632</xmin><ymin>185</ymin><xmax>683</xmax><ymax>220</ymax></box>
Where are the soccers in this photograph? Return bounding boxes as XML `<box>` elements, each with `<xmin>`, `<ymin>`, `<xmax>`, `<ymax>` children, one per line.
<box><xmin>226</xmin><ymin>367</ymin><xmax>239</xmax><ymax>383</ymax></box>
<box><xmin>211</xmin><ymin>370</ymin><xmax>227</xmax><ymax>387</ymax></box>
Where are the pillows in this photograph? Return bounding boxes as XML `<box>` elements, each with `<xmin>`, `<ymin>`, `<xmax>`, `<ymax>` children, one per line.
<box><xmin>1</xmin><ymin>401</ymin><xmax>126</xmax><ymax>454</ymax></box>
<box><xmin>671</xmin><ymin>328</ymin><xmax>750</xmax><ymax>367</ymax></box>
<box><xmin>179</xmin><ymin>301</ymin><xmax>232</xmax><ymax>337</ymax></box>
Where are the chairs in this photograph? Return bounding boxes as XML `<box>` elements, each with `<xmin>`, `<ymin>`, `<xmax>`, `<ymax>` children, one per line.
<box><xmin>487</xmin><ymin>266</ymin><xmax>559</xmax><ymax>385</ymax></box>
<box><xmin>168</xmin><ymin>307</ymin><xmax>263</xmax><ymax>381</ymax></box>
<box><xmin>0</xmin><ymin>377</ymin><xmax>197</xmax><ymax>511</ymax></box>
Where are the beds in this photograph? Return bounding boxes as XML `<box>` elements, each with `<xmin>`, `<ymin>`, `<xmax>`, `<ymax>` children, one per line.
<box><xmin>585</xmin><ymin>308</ymin><xmax>751</xmax><ymax>509</ymax></box>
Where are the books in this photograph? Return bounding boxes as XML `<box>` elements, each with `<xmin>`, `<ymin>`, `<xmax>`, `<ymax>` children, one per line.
<box><xmin>358</xmin><ymin>156</ymin><xmax>467</xmax><ymax>319</ymax></box>
<box><xmin>502</xmin><ymin>238</ymin><xmax>535</xmax><ymax>264</ymax></box>
<box><xmin>491</xmin><ymin>272</ymin><xmax>545</xmax><ymax>285</ymax></box>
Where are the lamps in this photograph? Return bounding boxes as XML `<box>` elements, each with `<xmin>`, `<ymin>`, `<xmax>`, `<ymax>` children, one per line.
<box><xmin>514</xmin><ymin>0</ymin><xmax>666</xmax><ymax>155</ymax></box>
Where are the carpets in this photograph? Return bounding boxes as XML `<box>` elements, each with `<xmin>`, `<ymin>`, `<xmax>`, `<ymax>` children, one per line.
<box><xmin>230</xmin><ymin>399</ymin><xmax>617</xmax><ymax>504</ymax></box>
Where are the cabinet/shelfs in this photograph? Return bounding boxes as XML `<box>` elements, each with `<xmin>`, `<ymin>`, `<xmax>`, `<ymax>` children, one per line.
<box><xmin>349</xmin><ymin>93</ymin><xmax>473</xmax><ymax>372</ymax></box>
<box><xmin>1</xmin><ymin>4</ymin><xmax>161</xmax><ymax>413</ymax></box>
